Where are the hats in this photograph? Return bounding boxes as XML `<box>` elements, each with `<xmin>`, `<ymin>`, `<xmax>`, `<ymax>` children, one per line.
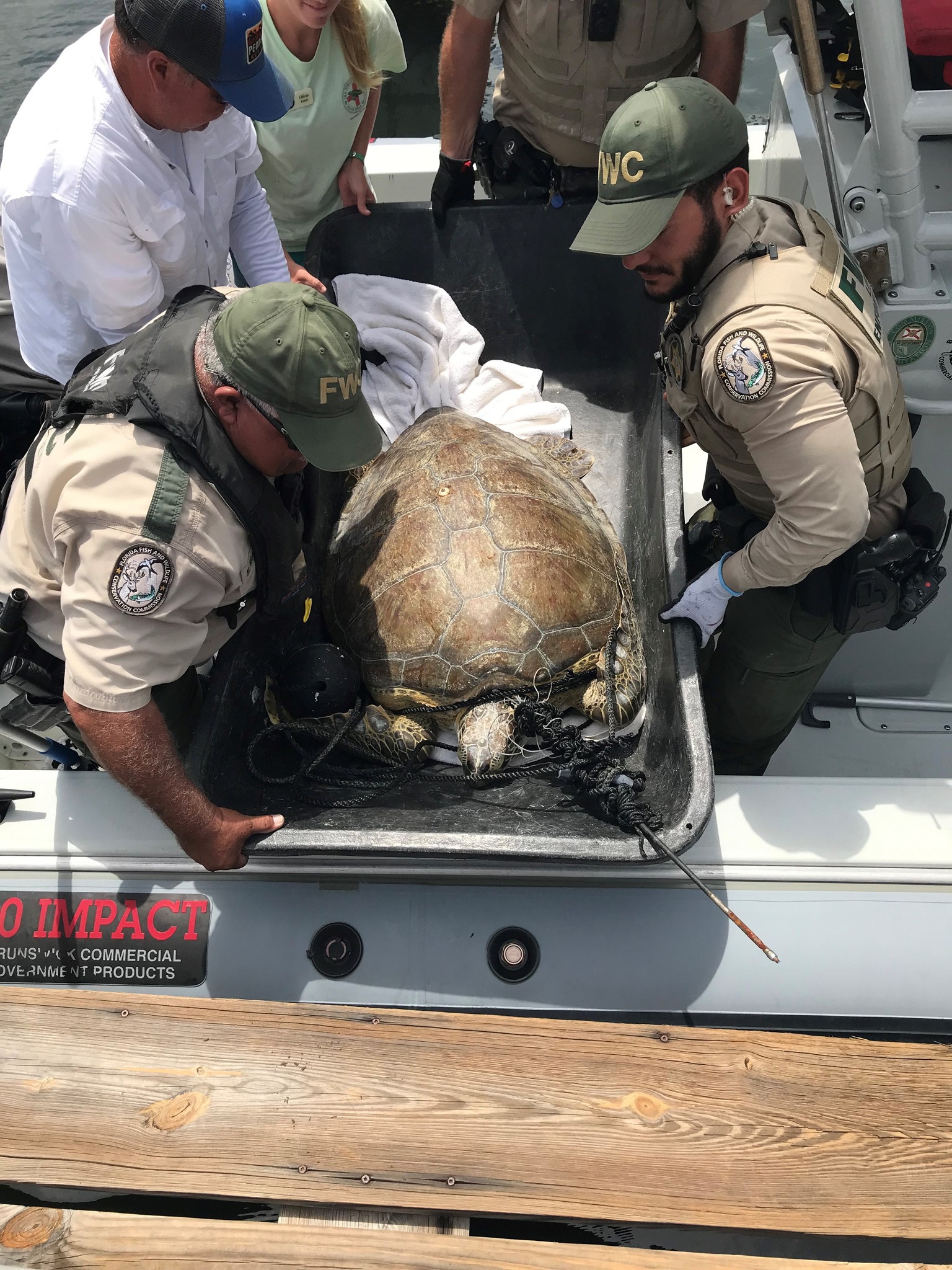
<box><xmin>569</xmin><ymin>77</ymin><xmax>749</xmax><ymax>257</ymax></box>
<box><xmin>215</xmin><ymin>281</ymin><xmax>383</xmax><ymax>473</ymax></box>
<box><xmin>123</xmin><ymin>0</ymin><xmax>295</xmax><ymax>122</ymax></box>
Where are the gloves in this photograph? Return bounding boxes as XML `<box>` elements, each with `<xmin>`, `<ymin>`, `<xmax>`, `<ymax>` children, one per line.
<box><xmin>430</xmin><ymin>151</ymin><xmax>477</xmax><ymax>230</ymax></box>
<box><xmin>657</xmin><ymin>551</ymin><xmax>745</xmax><ymax>662</ymax></box>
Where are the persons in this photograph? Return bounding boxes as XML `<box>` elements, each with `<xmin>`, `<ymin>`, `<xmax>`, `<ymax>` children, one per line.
<box><xmin>0</xmin><ymin>0</ymin><xmax>292</xmax><ymax>388</ymax></box>
<box><xmin>429</xmin><ymin>0</ymin><xmax>770</xmax><ymax>230</ymax></box>
<box><xmin>0</xmin><ymin>280</ymin><xmax>384</xmax><ymax>872</ymax></box>
<box><xmin>570</xmin><ymin>76</ymin><xmax>911</xmax><ymax>775</ymax></box>
<box><xmin>230</xmin><ymin>0</ymin><xmax>408</xmax><ymax>288</ymax></box>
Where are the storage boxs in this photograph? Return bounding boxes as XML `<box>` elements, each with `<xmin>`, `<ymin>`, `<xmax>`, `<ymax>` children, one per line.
<box><xmin>190</xmin><ymin>199</ymin><xmax>712</xmax><ymax>857</ymax></box>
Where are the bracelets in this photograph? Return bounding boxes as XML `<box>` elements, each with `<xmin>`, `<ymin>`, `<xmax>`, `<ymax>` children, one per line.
<box><xmin>348</xmin><ymin>151</ymin><xmax>365</xmax><ymax>164</ymax></box>
